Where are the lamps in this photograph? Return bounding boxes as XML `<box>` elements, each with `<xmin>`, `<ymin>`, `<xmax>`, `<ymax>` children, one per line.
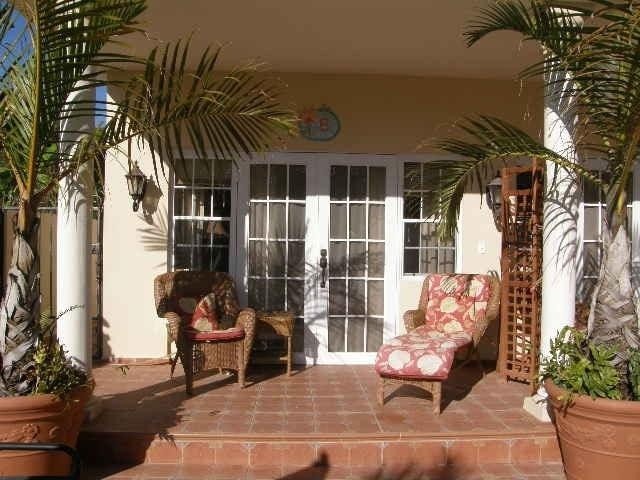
<box><xmin>486</xmin><ymin>169</ymin><xmax>502</xmax><ymax>209</ymax></box>
<box><xmin>125</xmin><ymin>160</ymin><xmax>150</xmax><ymax>212</ymax></box>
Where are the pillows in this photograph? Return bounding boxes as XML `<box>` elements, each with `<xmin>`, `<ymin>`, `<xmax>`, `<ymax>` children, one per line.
<box><xmin>171</xmin><ymin>290</ymin><xmax>202</xmax><ymax>326</ymax></box>
<box><xmin>189</xmin><ymin>290</ymin><xmax>219</xmax><ymax>334</ymax></box>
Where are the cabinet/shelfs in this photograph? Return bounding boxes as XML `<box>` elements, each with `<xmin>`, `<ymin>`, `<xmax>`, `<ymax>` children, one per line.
<box><xmin>496</xmin><ymin>153</ymin><xmax>545</xmax><ymax>396</ymax></box>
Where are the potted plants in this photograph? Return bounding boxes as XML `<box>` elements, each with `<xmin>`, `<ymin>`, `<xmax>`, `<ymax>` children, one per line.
<box><xmin>398</xmin><ymin>0</ymin><xmax>640</xmax><ymax>479</ymax></box>
<box><xmin>0</xmin><ymin>0</ymin><xmax>308</xmax><ymax>479</ymax></box>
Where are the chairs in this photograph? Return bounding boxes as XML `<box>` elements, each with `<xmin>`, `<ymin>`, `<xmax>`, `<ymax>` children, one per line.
<box><xmin>154</xmin><ymin>271</ymin><xmax>256</xmax><ymax>395</ymax></box>
<box><xmin>374</xmin><ymin>272</ymin><xmax>501</xmax><ymax>415</ymax></box>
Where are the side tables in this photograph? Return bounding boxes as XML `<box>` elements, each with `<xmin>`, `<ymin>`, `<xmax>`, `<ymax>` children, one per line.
<box><xmin>253</xmin><ymin>310</ymin><xmax>296</xmax><ymax>377</ymax></box>
